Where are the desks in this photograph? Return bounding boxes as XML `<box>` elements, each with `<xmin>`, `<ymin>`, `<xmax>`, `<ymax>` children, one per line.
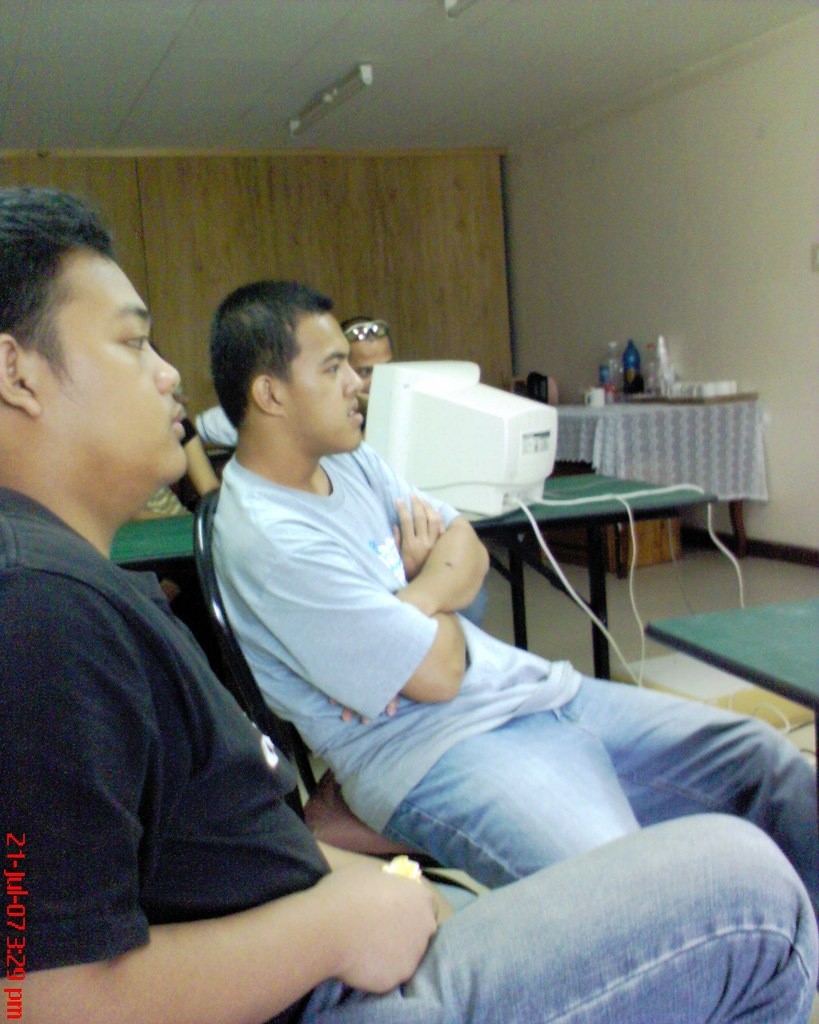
<box><xmin>537</xmin><ymin>391</ymin><xmax>761</xmax><ymax>579</ymax></box>
<box><xmin>114</xmin><ymin>472</ymin><xmax>717</xmax><ymax>750</ymax></box>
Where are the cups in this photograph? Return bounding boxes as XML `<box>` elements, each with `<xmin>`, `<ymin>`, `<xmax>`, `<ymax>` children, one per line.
<box><xmin>585</xmin><ymin>388</ymin><xmax>604</xmax><ymax>407</ymax></box>
<box><xmin>660</xmin><ymin>372</ymin><xmax>675</xmax><ymax>398</ymax></box>
<box><xmin>595</xmin><ymin>385</ymin><xmax>615</xmax><ymax>404</ymax></box>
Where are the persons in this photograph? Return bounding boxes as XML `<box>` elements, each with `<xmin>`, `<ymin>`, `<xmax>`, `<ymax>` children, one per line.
<box><xmin>0</xmin><ymin>185</ymin><xmax>818</xmax><ymax>1024</ymax></box>
<box><xmin>188</xmin><ymin>317</ymin><xmax>488</xmax><ymax>627</ymax></box>
<box><xmin>118</xmin><ymin>418</ymin><xmax>221</xmax><ymax>606</ymax></box>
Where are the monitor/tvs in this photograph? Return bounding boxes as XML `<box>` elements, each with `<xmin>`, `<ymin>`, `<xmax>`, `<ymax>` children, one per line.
<box><xmin>364</xmin><ymin>360</ymin><xmax>558</xmax><ymax>522</ymax></box>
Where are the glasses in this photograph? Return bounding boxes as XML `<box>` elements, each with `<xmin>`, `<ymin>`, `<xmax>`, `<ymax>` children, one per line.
<box><xmin>344</xmin><ymin>319</ymin><xmax>391</xmax><ymax>344</ymax></box>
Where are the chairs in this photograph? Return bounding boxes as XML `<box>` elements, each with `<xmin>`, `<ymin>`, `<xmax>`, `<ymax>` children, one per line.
<box><xmin>167</xmin><ymin>455</ymin><xmax>483</xmax><ymax>900</ymax></box>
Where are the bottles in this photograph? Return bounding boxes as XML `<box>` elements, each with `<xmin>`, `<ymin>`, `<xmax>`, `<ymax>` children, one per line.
<box><xmin>619</xmin><ymin>354</ymin><xmax>624</xmax><ymax>394</ymax></box>
<box><xmin>657</xmin><ymin>334</ymin><xmax>671</xmax><ymax>397</ymax></box>
<box><xmin>603</xmin><ymin>341</ymin><xmax>623</xmax><ymax>403</ymax></box>
<box><xmin>624</xmin><ymin>340</ymin><xmax>641</xmax><ymax>394</ymax></box>
<box><xmin>644</xmin><ymin>344</ymin><xmax>657</xmax><ymax>393</ymax></box>
<box><xmin>598</xmin><ymin>354</ymin><xmax>610</xmax><ymax>385</ymax></box>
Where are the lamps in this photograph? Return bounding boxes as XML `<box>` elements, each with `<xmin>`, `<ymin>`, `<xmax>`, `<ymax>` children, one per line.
<box><xmin>289</xmin><ymin>65</ymin><xmax>374</xmax><ymax>140</ymax></box>
